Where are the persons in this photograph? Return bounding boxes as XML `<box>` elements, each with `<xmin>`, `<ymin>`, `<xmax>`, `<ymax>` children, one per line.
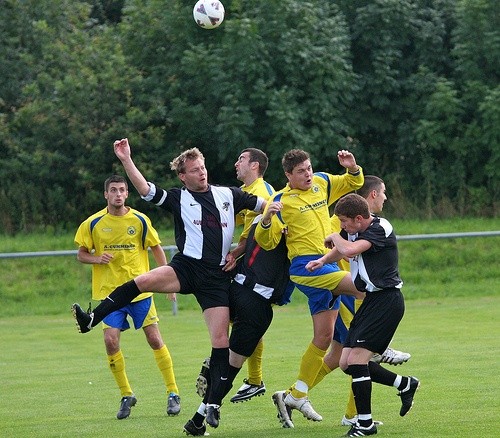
<box><xmin>306</xmin><ymin>193</ymin><xmax>420</xmax><ymax>438</ymax></box>
<box><xmin>255</xmin><ymin>149</ymin><xmax>412</xmax><ymax>422</ymax></box>
<box><xmin>73</xmin><ymin>138</ymin><xmax>268</xmax><ymax>428</ymax></box>
<box><xmin>232</xmin><ymin>148</ymin><xmax>295</xmax><ymax>402</ymax></box>
<box><xmin>183</xmin><ymin>223</ymin><xmax>289</xmax><ymax>437</ymax></box>
<box><xmin>272</xmin><ymin>175</ymin><xmax>388</xmax><ymax>429</ymax></box>
<box><xmin>74</xmin><ymin>176</ymin><xmax>181</xmax><ymax>420</ymax></box>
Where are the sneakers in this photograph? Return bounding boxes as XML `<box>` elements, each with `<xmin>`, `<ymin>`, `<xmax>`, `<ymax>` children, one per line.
<box><xmin>342</xmin><ymin>421</ymin><xmax>377</xmax><ymax>437</ymax></box>
<box><xmin>117</xmin><ymin>396</ymin><xmax>137</xmax><ymax>420</ymax></box>
<box><xmin>182</xmin><ymin>420</ymin><xmax>206</xmax><ymax>437</ymax></box>
<box><xmin>271</xmin><ymin>391</ymin><xmax>294</xmax><ymax>429</ymax></box>
<box><xmin>397</xmin><ymin>376</ymin><xmax>420</xmax><ymax>417</ymax></box>
<box><xmin>369</xmin><ymin>348</ymin><xmax>411</xmax><ymax>367</ymax></box>
<box><xmin>167</xmin><ymin>393</ymin><xmax>181</xmax><ymax>417</ymax></box>
<box><xmin>342</xmin><ymin>415</ymin><xmax>383</xmax><ymax>427</ymax></box>
<box><xmin>71</xmin><ymin>302</ymin><xmax>94</xmax><ymax>334</ymax></box>
<box><xmin>284</xmin><ymin>392</ymin><xmax>323</xmax><ymax>422</ymax></box>
<box><xmin>196</xmin><ymin>357</ymin><xmax>211</xmax><ymax>398</ymax></box>
<box><xmin>230</xmin><ymin>379</ymin><xmax>266</xmax><ymax>403</ymax></box>
<box><xmin>204</xmin><ymin>403</ymin><xmax>221</xmax><ymax>429</ymax></box>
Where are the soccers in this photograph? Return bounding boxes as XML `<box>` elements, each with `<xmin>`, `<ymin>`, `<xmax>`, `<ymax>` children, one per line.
<box><xmin>193</xmin><ymin>0</ymin><xmax>225</xmax><ymax>30</ymax></box>
